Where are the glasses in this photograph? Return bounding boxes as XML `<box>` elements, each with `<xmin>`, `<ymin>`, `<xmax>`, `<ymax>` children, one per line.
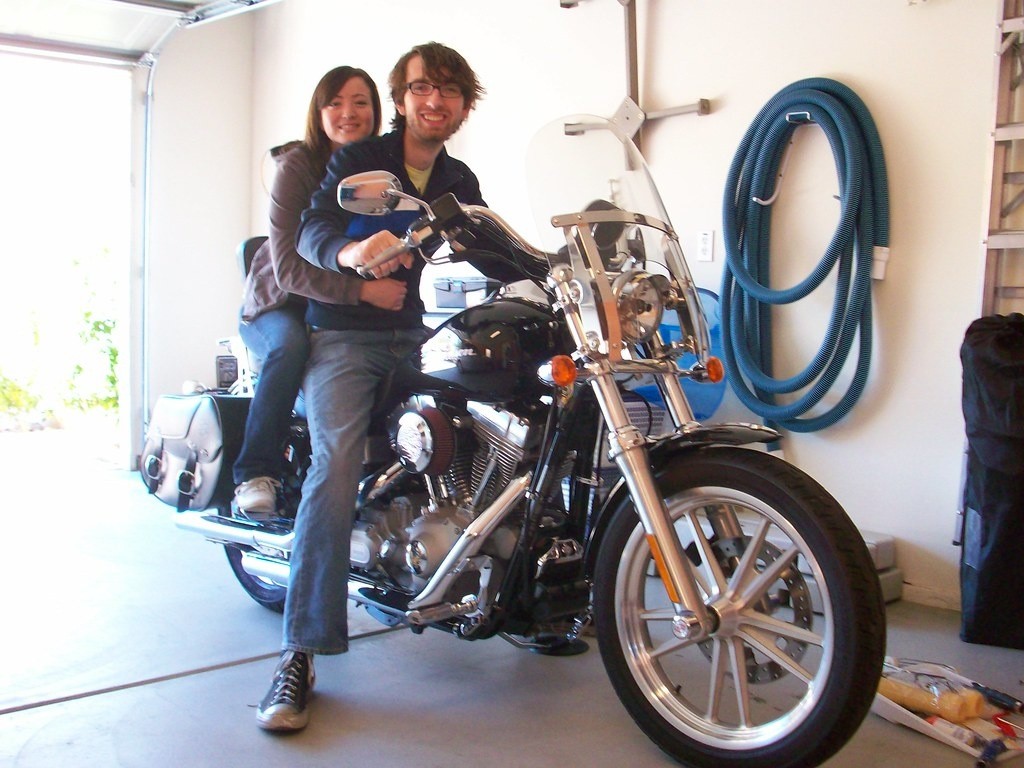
<box><xmin>401</xmin><ymin>76</ymin><xmax>474</xmax><ymax>96</ymax></box>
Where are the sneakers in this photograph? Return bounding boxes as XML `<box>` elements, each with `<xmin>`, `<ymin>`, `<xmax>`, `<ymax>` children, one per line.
<box><xmin>536</xmin><ymin>609</ymin><xmax>599</xmax><ymax>643</ymax></box>
<box><xmin>256</xmin><ymin>643</ymin><xmax>321</xmax><ymax>733</ymax></box>
<box><xmin>233</xmin><ymin>473</ymin><xmax>282</xmax><ymax>517</ymax></box>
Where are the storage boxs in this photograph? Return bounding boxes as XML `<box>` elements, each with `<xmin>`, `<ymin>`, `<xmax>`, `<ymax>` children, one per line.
<box><xmin>643</xmin><ymin>514</ymin><xmax>904</xmax><ymax>617</ymax></box>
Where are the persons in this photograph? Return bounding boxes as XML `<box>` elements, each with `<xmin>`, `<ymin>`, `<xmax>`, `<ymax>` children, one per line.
<box><xmin>256</xmin><ymin>41</ymin><xmax>595</xmax><ymax>731</ymax></box>
<box><xmin>233</xmin><ymin>67</ymin><xmax>407</xmax><ymax>514</ymax></box>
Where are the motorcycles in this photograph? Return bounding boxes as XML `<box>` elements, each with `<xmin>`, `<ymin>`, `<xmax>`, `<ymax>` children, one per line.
<box><xmin>172</xmin><ymin>112</ymin><xmax>887</xmax><ymax>768</ymax></box>
